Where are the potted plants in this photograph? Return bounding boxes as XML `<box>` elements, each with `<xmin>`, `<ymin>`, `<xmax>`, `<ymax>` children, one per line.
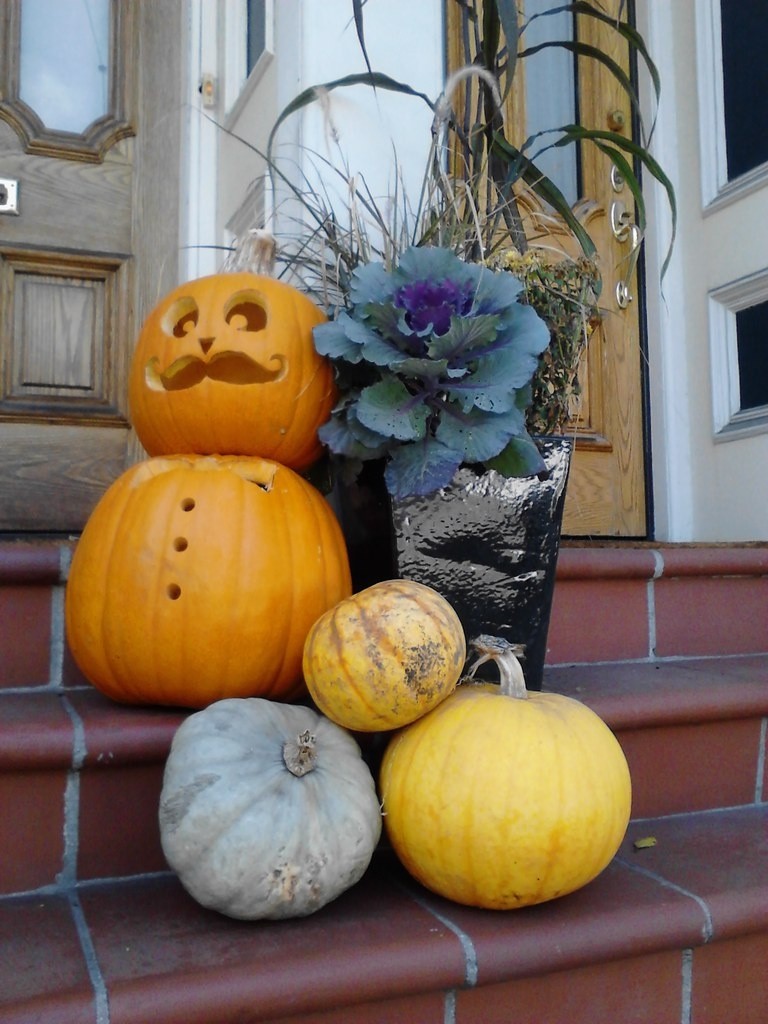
<box><xmin>192</xmin><ymin>0</ymin><xmax>677</xmax><ymax>687</ymax></box>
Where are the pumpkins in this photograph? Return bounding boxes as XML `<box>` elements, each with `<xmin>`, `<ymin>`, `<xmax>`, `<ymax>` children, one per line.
<box><xmin>66</xmin><ymin>269</ymin><xmax>630</xmax><ymax>919</ymax></box>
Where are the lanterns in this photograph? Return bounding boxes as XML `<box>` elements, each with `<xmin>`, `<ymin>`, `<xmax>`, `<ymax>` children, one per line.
<box><xmin>65</xmin><ymin>456</ymin><xmax>352</xmax><ymax>708</ymax></box>
<box><xmin>127</xmin><ymin>230</ymin><xmax>337</xmax><ymax>469</ymax></box>
